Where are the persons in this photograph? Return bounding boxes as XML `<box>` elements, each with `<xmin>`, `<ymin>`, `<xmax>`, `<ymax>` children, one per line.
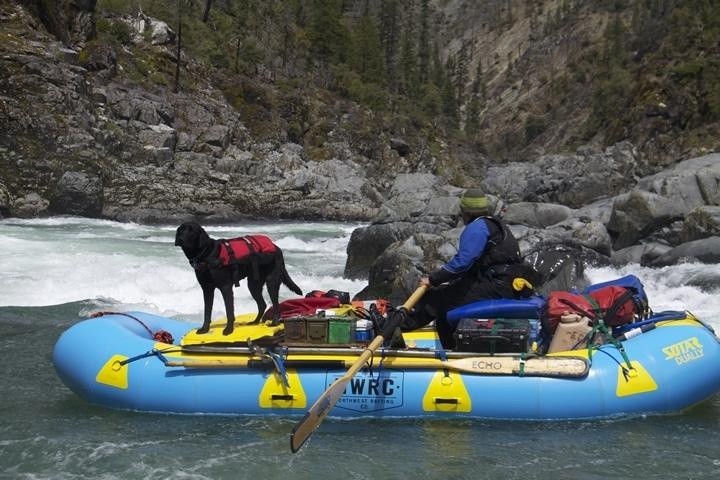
<box><xmin>370</xmin><ymin>189</ymin><xmax>533</xmax><ymax>348</ymax></box>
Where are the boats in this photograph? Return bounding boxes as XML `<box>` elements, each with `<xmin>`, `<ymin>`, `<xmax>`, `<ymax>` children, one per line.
<box><xmin>52</xmin><ymin>305</ymin><xmax>716</xmax><ymax>416</ymax></box>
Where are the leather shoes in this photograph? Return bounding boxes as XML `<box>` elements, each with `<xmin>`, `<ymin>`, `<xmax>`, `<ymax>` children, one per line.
<box><xmin>370</xmin><ymin>304</ymin><xmax>388</xmax><ymax>335</ymax></box>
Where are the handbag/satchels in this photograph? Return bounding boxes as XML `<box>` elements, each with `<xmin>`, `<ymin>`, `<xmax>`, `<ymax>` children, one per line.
<box><xmin>545</xmin><ymin>285</ymin><xmax>634</xmax><ymax>335</ymax></box>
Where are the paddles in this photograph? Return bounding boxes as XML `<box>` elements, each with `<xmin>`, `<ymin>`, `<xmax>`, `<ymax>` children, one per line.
<box><xmin>290</xmin><ymin>286</ymin><xmax>428</xmax><ymax>453</ymax></box>
<box><xmin>183</xmin><ymin>357</ymin><xmax>590</xmax><ymax>378</ymax></box>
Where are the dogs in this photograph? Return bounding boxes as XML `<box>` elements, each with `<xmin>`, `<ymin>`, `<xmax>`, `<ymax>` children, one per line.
<box><xmin>174</xmin><ymin>221</ymin><xmax>303</xmax><ymax>337</ymax></box>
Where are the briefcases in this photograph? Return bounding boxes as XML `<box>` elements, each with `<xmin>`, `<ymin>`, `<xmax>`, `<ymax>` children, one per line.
<box><xmin>452</xmin><ymin>318</ymin><xmax>531</xmax><ymax>354</ymax></box>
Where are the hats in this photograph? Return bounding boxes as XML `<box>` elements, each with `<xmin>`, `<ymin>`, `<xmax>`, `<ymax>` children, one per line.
<box><xmin>460</xmin><ymin>189</ymin><xmax>490</xmax><ymax>215</ymax></box>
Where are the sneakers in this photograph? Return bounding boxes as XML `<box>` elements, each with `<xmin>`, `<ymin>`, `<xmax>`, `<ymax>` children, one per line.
<box><xmin>386</xmin><ymin>303</ymin><xmax>394</xmax><ymax>317</ymax></box>
